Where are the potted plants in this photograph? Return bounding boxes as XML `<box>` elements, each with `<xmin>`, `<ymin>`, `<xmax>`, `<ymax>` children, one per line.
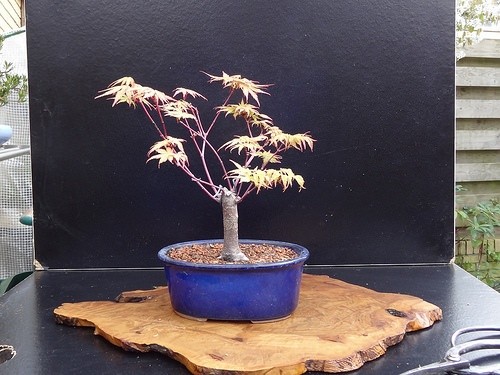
<box><xmin>93</xmin><ymin>69</ymin><xmax>318</xmax><ymax>323</ymax></box>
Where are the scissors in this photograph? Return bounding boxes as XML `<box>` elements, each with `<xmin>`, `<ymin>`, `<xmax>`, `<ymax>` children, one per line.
<box><xmin>390</xmin><ymin>324</ymin><xmax>500</xmax><ymax>375</ymax></box>
